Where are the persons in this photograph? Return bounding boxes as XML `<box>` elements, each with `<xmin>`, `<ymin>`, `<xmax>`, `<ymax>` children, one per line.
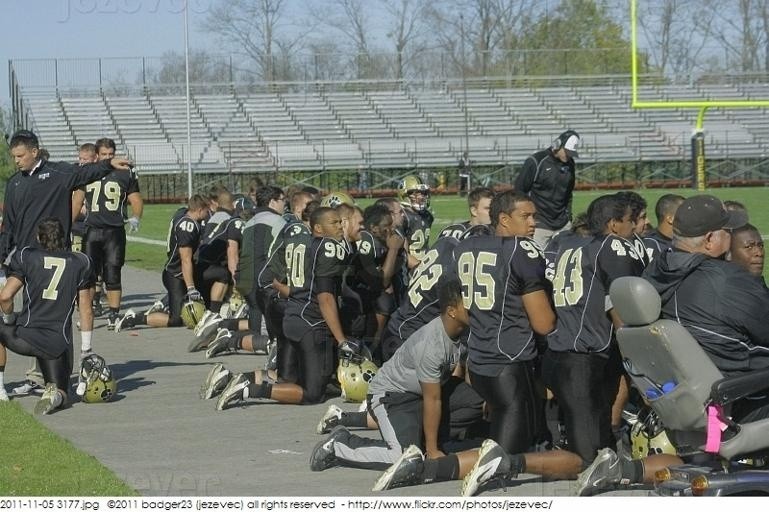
<box><xmin>71</xmin><ymin>138</ymin><xmax>144</xmax><ymax>333</ymax></box>
<box><xmin>459</xmin><ymin>152</ymin><xmax>472</xmax><ymax>192</ymax></box>
<box><xmin>71</xmin><ymin>142</ymin><xmax>104</xmax><ymax>318</ymax></box>
<box><xmin>513</xmin><ymin>131</ymin><xmax>580</xmax><ymax>260</ymax></box>
<box><xmin>114</xmin><ymin>178</ymin><xmax>769</xmax><ymax>496</ymax></box>
<box><xmin>0</xmin><ymin>216</ymin><xmax>110</xmax><ymax>415</ymax></box>
<box><xmin>38</xmin><ymin>146</ymin><xmax>51</xmax><ymax>163</ymax></box>
<box><xmin>1</xmin><ymin>129</ymin><xmax>136</xmax><ymax>400</ymax></box>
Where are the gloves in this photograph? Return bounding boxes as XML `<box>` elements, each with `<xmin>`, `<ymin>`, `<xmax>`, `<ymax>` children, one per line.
<box><xmin>2</xmin><ymin>314</ymin><xmax>18</xmax><ymax>325</ymax></box>
<box><xmin>186</xmin><ymin>286</ymin><xmax>203</xmax><ymax>301</ymax></box>
<box><xmin>123</xmin><ymin>215</ymin><xmax>142</xmax><ymax>234</ymax></box>
<box><xmin>337</xmin><ymin>336</ymin><xmax>364</xmax><ymax>362</ymax></box>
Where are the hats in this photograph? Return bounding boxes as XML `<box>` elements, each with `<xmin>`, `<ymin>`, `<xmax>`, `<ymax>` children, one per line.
<box><xmin>557</xmin><ymin>130</ymin><xmax>581</xmax><ymax>159</ymax></box>
<box><xmin>672</xmin><ymin>194</ymin><xmax>751</xmax><ymax>238</ymax></box>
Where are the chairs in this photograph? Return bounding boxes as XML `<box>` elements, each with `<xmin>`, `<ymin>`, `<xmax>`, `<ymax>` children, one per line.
<box><xmin>608</xmin><ymin>276</ymin><xmax>769</xmax><ymax>463</ymax></box>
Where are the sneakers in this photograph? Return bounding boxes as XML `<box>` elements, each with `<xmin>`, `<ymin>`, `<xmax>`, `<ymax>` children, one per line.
<box><xmin>186</xmin><ymin>325</ymin><xmax>220</xmax><ymax>355</ymax></box>
<box><xmin>144</xmin><ymin>300</ymin><xmax>165</xmax><ymax>316</ymax></box>
<box><xmin>114</xmin><ymin>307</ymin><xmax>137</xmax><ymax>334</ymax></box>
<box><xmin>311</xmin><ymin>403</ymin><xmax>351</xmax><ymax>473</ymax></box>
<box><xmin>576</xmin><ymin>446</ymin><xmax>623</xmax><ymax>497</ymax></box>
<box><xmin>12</xmin><ymin>376</ymin><xmax>65</xmax><ymax>417</ymax></box>
<box><xmin>192</xmin><ymin>303</ymin><xmax>249</xmax><ymax>337</ymax></box>
<box><xmin>372</xmin><ymin>443</ymin><xmax>425</xmax><ymax>494</ymax></box>
<box><xmin>198</xmin><ymin>361</ymin><xmax>230</xmax><ymax>400</ymax></box>
<box><xmin>216</xmin><ymin>371</ymin><xmax>253</xmax><ymax>410</ymax></box>
<box><xmin>76</xmin><ymin>305</ymin><xmax>104</xmax><ymax>331</ymax></box>
<box><xmin>205</xmin><ymin>328</ymin><xmax>231</xmax><ymax>359</ymax></box>
<box><xmin>107</xmin><ymin>312</ymin><xmax>120</xmax><ymax>330</ymax></box>
<box><xmin>460</xmin><ymin>436</ymin><xmax>510</xmax><ymax>497</ymax></box>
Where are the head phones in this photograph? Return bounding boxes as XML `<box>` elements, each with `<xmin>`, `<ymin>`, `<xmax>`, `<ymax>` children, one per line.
<box><xmin>553</xmin><ymin>131</ymin><xmax>581</xmax><ymax>151</ymax></box>
<box><xmin>12</xmin><ymin>131</ymin><xmax>37</xmax><ymax>144</ymax></box>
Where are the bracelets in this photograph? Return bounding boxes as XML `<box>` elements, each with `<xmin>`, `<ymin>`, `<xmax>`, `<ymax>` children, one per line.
<box><xmin>81</xmin><ymin>331</ymin><xmax>93</xmax><ymax>354</ymax></box>
<box><xmin>2</xmin><ymin>311</ymin><xmax>17</xmax><ymax>327</ymax></box>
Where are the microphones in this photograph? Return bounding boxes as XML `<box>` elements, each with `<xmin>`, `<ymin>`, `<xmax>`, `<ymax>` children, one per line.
<box><xmin>4</xmin><ymin>134</ymin><xmax>10</xmax><ymax>149</ymax></box>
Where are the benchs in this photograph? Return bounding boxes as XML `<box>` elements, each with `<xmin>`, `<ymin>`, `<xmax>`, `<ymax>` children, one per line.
<box><xmin>20</xmin><ymin>77</ymin><xmax>767</xmax><ymax>174</ymax></box>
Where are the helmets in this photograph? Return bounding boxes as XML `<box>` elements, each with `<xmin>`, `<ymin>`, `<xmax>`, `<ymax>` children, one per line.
<box><xmin>228</xmin><ymin>287</ymin><xmax>246</xmax><ymax>313</ymax></box>
<box><xmin>396</xmin><ymin>175</ymin><xmax>431</xmax><ymax>212</ymax></box>
<box><xmin>629</xmin><ymin>416</ymin><xmax>678</xmax><ymax>461</ymax></box>
<box><xmin>319</xmin><ymin>192</ymin><xmax>354</xmax><ymax>210</ymax></box>
<box><xmin>179</xmin><ymin>298</ymin><xmax>208</xmax><ymax>330</ymax></box>
<box><xmin>335</xmin><ymin>357</ymin><xmax>379</xmax><ymax>404</ymax></box>
<box><xmin>76</xmin><ymin>356</ymin><xmax>117</xmax><ymax>405</ymax></box>
